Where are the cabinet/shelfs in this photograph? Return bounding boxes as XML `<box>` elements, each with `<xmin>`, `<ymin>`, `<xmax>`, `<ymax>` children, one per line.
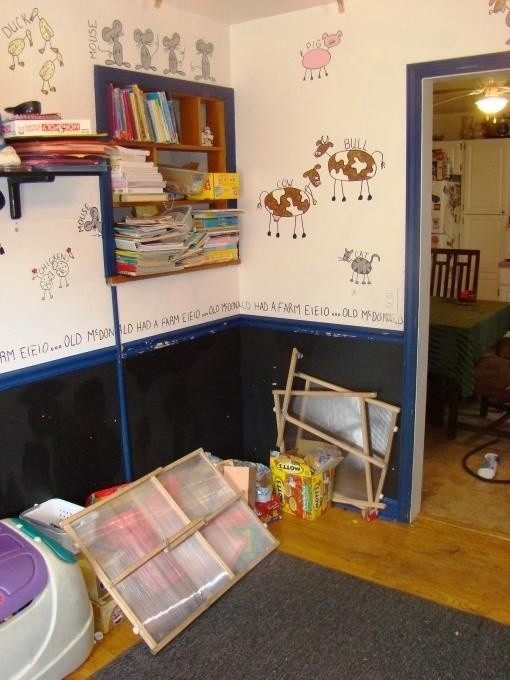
<box><xmin>461</xmin><ymin>138</ymin><xmax>510</xmax><ymax>301</ymax></box>
<box><xmin>94</xmin><ymin>65</ymin><xmax>241</xmax><ymax>284</ymax></box>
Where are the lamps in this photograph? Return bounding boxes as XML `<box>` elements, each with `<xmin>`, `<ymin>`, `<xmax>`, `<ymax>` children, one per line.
<box><xmin>474</xmin><ymin>91</ymin><xmax>508</xmax><ymax>124</ymax></box>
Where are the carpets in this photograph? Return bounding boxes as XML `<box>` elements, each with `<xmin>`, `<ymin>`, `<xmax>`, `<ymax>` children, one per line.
<box><xmin>81</xmin><ymin>549</ymin><xmax>510</xmax><ymax>680</ymax></box>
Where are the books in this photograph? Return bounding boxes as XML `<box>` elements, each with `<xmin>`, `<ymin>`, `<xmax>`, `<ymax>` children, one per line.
<box><xmin>105</xmin><ymin>82</ymin><xmax>243</xmax><ymax>279</ymax></box>
<box><xmin>0</xmin><ymin>111</ymin><xmax>116</xmax><ymax>176</ymax></box>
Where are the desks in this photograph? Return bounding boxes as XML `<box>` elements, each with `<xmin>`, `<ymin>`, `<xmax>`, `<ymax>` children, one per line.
<box><xmin>429</xmin><ymin>296</ymin><xmax>510</xmax><ymax>439</ymax></box>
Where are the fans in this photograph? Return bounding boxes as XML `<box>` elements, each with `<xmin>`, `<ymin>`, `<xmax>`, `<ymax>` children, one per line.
<box><xmin>433</xmin><ymin>78</ymin><xmax>510</xmax><ymax>106</ymax></box>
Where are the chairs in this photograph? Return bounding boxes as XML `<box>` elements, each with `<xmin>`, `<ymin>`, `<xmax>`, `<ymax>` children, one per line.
<box><xmin>430</xmin><ymin>248</ymin><xmax>480</xmax><ymax>297</ymax></box>
<box><xmin>448</xmin><ymin>336</ymin><xmax>510</xmax><ymax>439</ymax></box>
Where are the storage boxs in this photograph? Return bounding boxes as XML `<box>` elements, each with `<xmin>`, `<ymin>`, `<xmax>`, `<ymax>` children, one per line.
<box><xmin>80</xmin><ymin>551</ymin><xmax>132</xmax><ymax>632</ymax></box>
<box><xmin>269</xmin><ymin>439</ymin><xmax>345</xmax><ymax>522</ymax></box>
<box><xmin>158</xmin><ymin>164</ymin><xmax>240</xmax><ymax>200</ymax></box>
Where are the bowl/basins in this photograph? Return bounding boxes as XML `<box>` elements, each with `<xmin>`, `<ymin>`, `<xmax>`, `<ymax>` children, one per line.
<box><xmin>4</xmin><ymin>101</ymin><xmax>41</xmax><ymax>114</ymax></box>
